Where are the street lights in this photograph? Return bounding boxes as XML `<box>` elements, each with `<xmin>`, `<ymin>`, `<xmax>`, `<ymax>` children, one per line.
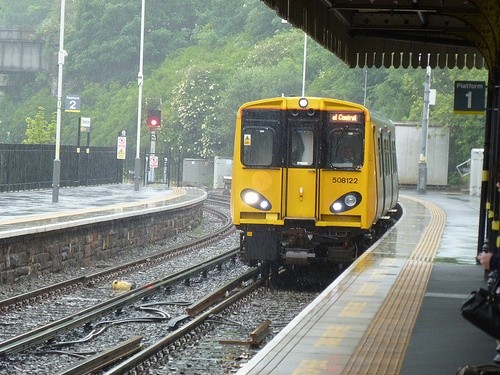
<box><xmin>282</xmin><ymin>18</ymin><xmax>307</xmax><ymax>97</ymax></box>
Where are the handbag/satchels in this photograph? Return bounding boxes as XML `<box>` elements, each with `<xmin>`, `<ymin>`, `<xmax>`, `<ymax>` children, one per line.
<box><xmin>457</xmin><ymin>362</ymin><xmax>500</xmax><ymax>375</ymax></box>
<box><xmin>460</xmin><ymin>278</ymin><xmax>500</xmax><ymax>341</ymax></box>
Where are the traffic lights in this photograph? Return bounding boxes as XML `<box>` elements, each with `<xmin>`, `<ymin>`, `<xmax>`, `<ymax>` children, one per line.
<box><xmin>147</xmin><ymin>110</ymin><xmax>161</xmax><ymax>128</ymax></box>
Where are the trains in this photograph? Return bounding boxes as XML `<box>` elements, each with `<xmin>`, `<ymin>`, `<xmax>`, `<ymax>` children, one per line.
<box><xmin>230</xmin><ymin>97</ymin><xmax>401</xmax><ymax>282</ymax></box>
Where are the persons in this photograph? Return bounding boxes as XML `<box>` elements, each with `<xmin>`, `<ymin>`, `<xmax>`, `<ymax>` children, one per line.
<box><xmin>477</xmin><ymin>175</ymin><xmax>500</xmax><ymax>363</ymax></box>
<box><xmin>339</xmin><ymin>146</ymin><xmax>358</xmax><ymax>165</ymax></box>
